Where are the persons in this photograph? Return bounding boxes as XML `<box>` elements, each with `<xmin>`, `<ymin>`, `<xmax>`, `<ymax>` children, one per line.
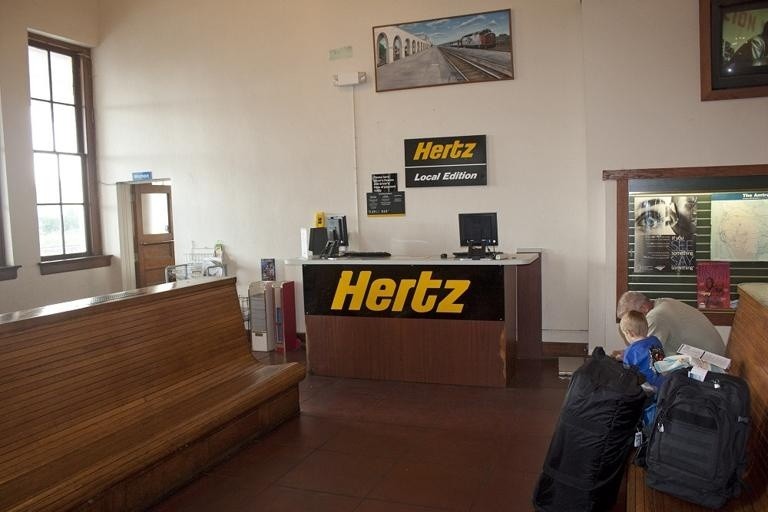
<box><xmin>616</xmin><ymin>291</ymin><xmax>727</xmax><ymax>362</ymax></box>
<box><xmin>635</xmin><ymin>196</ymin><xmax>677</xmax><ymax>235</ymax></box>
<box><xmin>619</xmin><ymin>311</ymin><xmax>665</xmax><ymax>402</ymax></box>
<box><xmin>673</xmin><ymin>195</ymin><xmax>697</xmax><ymax>232</ymax></box>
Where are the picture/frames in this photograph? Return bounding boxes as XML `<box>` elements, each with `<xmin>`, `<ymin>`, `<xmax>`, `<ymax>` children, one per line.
<box><xmin>697</xmin><ymin>1</ymin><xmax>768</xmax><ymax>102</ymax></box>
<box><xmin>371</xmin><ymin>9</ymin><xmax>515</xmax><ymax>92</ymax></box>
<box><xmin>603</xmin><ymin>163</ymin><xmax>768</xmax><ymax>324</ymax></box>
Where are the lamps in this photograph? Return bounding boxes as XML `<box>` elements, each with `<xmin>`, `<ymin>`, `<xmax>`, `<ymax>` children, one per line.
<box><xmin>331</xmin><ymin>71</ymin><xmax>366</xmax><ymax>87</ymax></box>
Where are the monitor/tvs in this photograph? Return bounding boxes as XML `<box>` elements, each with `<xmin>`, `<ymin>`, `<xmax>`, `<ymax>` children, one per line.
<box><xmin>711</xmin><ymin>0</ymin><xmax>768</xmax><ymax>90</ymax></box>
<box><xmin>319</xmin><ymin>214</ymin><xmax>352</xmax><ymax>258</ymax></box>
<box><xmin>458</xmin><ymin>213</ymin><xmax>497</xmax><ymax>258</ymax></box>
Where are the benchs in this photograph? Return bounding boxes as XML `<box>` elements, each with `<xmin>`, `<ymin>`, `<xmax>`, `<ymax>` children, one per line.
<box><xmin>624</xmin><ymin>284</ymin><xmax>768</xmax><ymax>512</ymax></box>
<box><xmin>0</xmin><ymin>275</ymin><xmax>303</xmax><ymax>511</ymax></box>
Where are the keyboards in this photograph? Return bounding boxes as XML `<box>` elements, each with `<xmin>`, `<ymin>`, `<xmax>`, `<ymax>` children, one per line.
<box><xmin>454</xmin><ymin>252</ymin><xmax>503</xmax><ymax>256</ymax></box>
<box><xmin>343</xmin><ymin>252</ymin><xmax>392</xmax><ymax>257</ymax></box>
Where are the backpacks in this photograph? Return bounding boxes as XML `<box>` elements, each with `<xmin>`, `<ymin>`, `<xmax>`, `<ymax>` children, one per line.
<box><xmin>532</xmin><ymin>347</ymin><xmax>655</xmax><ymax>512</ymax></box>
<box><xmin>630</xmin><ymin>365</ymin><xmax>752</xmax><ymax>510</ymax></box>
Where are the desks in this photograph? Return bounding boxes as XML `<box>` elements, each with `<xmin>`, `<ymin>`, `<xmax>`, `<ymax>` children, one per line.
<box><xmin>302</xmin><ymin>252</ymin><xmax>541</xmax><ymax>389</ymax></box>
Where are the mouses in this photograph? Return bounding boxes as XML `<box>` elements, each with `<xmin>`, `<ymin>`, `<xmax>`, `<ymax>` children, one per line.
<box><xmin>441</xmin><ymin>254</ymin><xmax>447</xmax><ymax>258</ymax></box>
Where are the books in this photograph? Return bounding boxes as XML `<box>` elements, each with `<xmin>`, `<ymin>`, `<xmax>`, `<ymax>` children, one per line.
<box><xmin>695</xmin><ymin>261</ymin><xmax>731</xmax><ymax>309</ymax></box>
